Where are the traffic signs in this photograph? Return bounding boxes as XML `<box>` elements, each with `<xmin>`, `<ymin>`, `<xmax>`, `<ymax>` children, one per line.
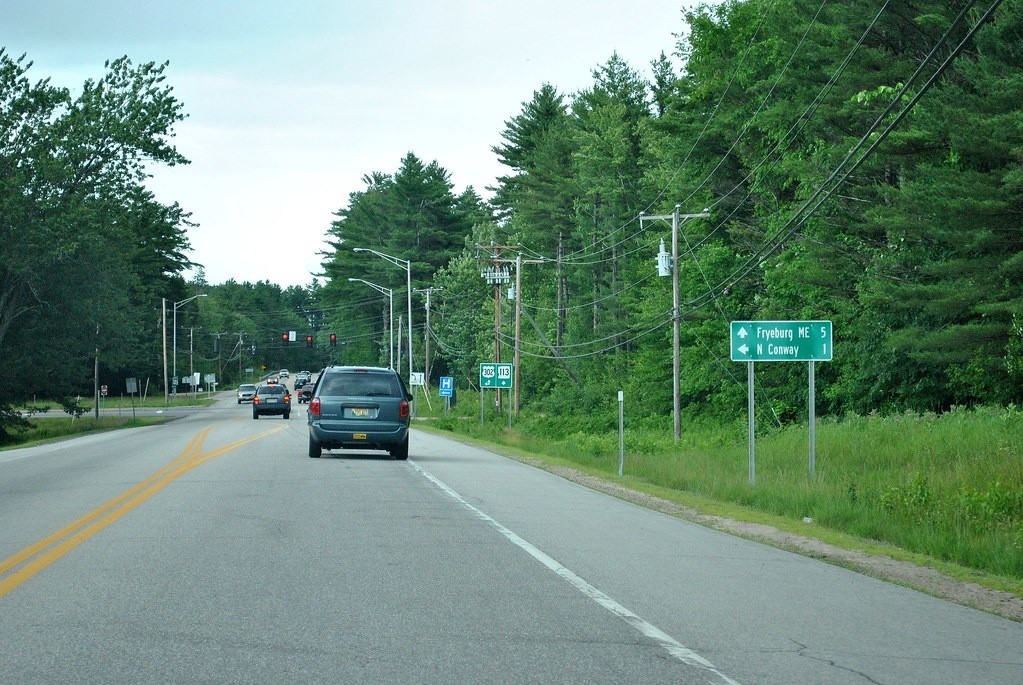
<box><xmin>480</xmin><ymin>362</ymin><xmax>496</xmax><ymax>388</ymax></box>
<box><xmin>730</xmin><ymin>321</ymin><xmax>834</xmax><ymax>362</ymax></box>
<box><xmin>496</xmin><ymin>363</ymin><xmax>513</xmax><ymax>388</ymax></box>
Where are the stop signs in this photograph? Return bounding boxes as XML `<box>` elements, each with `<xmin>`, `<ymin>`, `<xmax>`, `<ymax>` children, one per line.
<box><xmin>100</xmin><ymin>385</ymin><xmax>108</xmax><ymax>395</ymax></box>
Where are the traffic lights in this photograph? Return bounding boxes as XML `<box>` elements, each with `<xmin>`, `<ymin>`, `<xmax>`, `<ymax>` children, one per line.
<box><xmin>306</xmin><ymin>336</ymin><xmax>312</xmax><ymax>348</ymax></box>
<box><xmin>330</xmin><ymin>334</ymin><xmax>337</xmax><ymax>347</ymax></box>
<box><xmin>282</xmin><ymin>334</ymin><xmax>289</xmax><ymax>347</ymax></box>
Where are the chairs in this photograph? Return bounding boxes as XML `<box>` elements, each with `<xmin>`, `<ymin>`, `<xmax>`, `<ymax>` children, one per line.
<box><xmin>327</xmin><ymin>381</ymin><xmax>347</xmax><ymax>396</ymax></box>
<box><xmin>368</xmin><ymin>384</ymin><xmax>386</xmax><ymax>393</ymax></box>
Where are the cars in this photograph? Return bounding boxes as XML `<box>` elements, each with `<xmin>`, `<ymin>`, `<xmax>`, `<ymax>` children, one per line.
<box><xmin>298</xmin><ymin>382</ymin><xmax>316</xmax><ymax>404</ymax></box>
<box><xmin>300</xmin><ymin>371</ymin><xmax>313</xmax><ymax>382</ymax></box>
<box><xmin>277</xmin><ymin>369</ymin><xmax>290</xmax><ymax>378</ymax></box>
<box><xmin>296</xmin><ymin>373</ymin><xmax>310</xmax><ymax>384</ymax></box>
<box><xmin>236</xmin><ymin>384</ymin><xmax>258</xmax><ymax>403</ymax></box>
<box><xmin>306</xmin><ymin>365</ymin><xmax>414</xmax><ymax>460</ymax></box>
<box><xmin>252</xmin><ymin>383</ymin><xmax>292</xmax><ymax>419</ymax></box>
<box><xmin>294</xmin><ymin>377</ymin><xmax>307</xmax><ymax>391</ymax></box>
<box><xmin>267</xmin><ymin>376</ymin><xmax>279</xmax><ymax>385</ymax></box>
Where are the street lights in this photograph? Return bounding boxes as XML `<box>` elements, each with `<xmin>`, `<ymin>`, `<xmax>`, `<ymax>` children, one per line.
<box><xmin>352</xmin><ymin>247</ymin><xmax>414</xmax><ymax>414</ymax></box>
<box><xmin>347</xmin><ymin>276</ymin><xmax>393</xmax><ymax>370</ymax></box>
<box><xmin>172</xmin><ymin>293</ymin><xmax>209</xmax><ymax>399</ymax></box>
